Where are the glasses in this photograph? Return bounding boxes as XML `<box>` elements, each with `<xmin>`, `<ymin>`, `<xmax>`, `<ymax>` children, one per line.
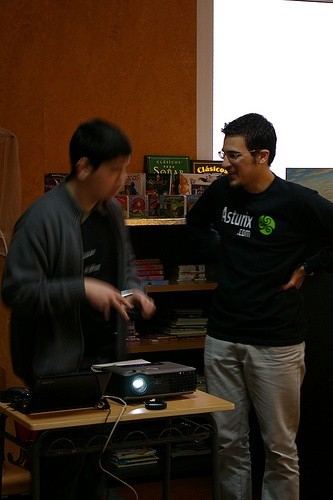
<box><xmin>217</xmin><ymin>149</ymin><xmax>261</xmax><ymax>161</ymax></box>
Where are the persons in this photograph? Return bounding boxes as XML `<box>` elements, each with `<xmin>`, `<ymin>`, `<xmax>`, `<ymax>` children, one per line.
<box><xmin>7</xmin><ymin>117</ymin><xmax>157</xmax><ymax>500</ymax></box>
<box><xmin>184</xmin><ymin>112</ymin><xmax>333</xmax><ymax>500</ymax></box>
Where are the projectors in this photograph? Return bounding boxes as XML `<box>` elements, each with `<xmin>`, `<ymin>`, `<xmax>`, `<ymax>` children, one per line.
<box><xmin>95</xmin><ymin>361</ymin><xmax>197</xmax><ymax>403</ymax></box>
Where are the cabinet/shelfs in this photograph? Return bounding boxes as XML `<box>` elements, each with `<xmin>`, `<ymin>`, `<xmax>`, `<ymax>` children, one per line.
<box><xmin>119</xmin><ymin>217</ymin><xmax>218</xmax><ymax>392</ymax></box>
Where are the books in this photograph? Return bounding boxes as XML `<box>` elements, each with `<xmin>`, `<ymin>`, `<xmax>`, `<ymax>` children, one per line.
<box><xmin>126</xmin><ymin>259</ymin><xmax>168</xmax><ymax>286</ymax></box>
<box><xmin>148</xmin><ymin>175</ymin><xmax>208</xmax><ymax>225</ymax></box>
<box><xmin>165</xmin><ymin>314</ymin><xmax>209</xmax><ymax>339</ymax></box>
<box><xmin>130</xmin><ymin>195</ymin><xmax>148</xmax><ymax>219</ymax></box>
<box><xmin>114</xmin><ymin>194</ymin><xmax>127</xmax><ymax>218</ymax></box>
<box><xmin>122</xmin><ymin>172</ymin><xmax>146</xmax><ymax>194</ymax></box>
<box><xmin>144</xmin><ymin>156</ymin><xmax>189</xmax><ymax>189</ymax></box>
<box><xmin>193</xmin><ymin>160</ymin><xmax>224</xmax><ymax>193</ymax></box>
<box><xmin>177</xmin><ymin>265</ymin><xmax>204</xmax><ymax>284</ymax></box>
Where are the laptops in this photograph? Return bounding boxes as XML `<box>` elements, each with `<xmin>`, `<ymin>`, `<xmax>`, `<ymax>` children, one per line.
<box><xmin>10</xmin><ymin>372</ymin><xmax>111</xmax><ymax>415</ymax></box>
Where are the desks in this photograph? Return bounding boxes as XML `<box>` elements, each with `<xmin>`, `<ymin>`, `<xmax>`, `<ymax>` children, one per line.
<box><xmin>0</xmin><ymin>390</ymin><xmax>235</xmax><ymax>500</ymax></box>
